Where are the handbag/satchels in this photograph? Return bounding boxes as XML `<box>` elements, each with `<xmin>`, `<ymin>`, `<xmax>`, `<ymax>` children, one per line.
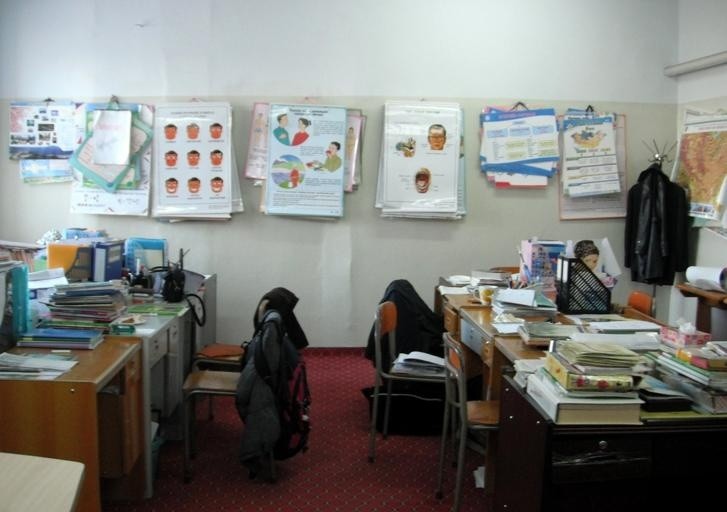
<box><xmin>151</xmin><ymin>262</ymin><xmax>206</xmax><ymax>327</ymax></box>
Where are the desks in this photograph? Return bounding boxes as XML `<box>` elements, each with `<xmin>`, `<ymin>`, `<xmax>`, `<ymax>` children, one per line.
<box><xmin>489</xmin><ymin>336</ymin><xmax>547</xmax><ymax>398</ymax></box>
<box><xmin>459</xmin><ymin>307</ymin><xmax>570</xmax><ymax>492</ymax></box>
<box><xmin>0</xmin><ymin>337</ymin><xmax>145</xmax><ymax>512</ymax></box>
<box><xmin>2</xmin><ymin>450</ymin><xmax>90</xmax><ymax>512</ymax></box>
<box><xmin>432</xmin><ymin>285</ymin><xmax>488</xmax><ymax>337</ymax></box>
<box><xmin>136</xmin><ymin>300</ymin><xmax>196</xmax><ymax>424</ymax></box>
<box><xmin>493</xmin><ymin>374</ymin><xmax>726</xmax><ymax>511</ymax></box>
<box><xmin>102</xmin><ymin>314</ymin><xmax>179</xmax><ymax>497</ymax></box>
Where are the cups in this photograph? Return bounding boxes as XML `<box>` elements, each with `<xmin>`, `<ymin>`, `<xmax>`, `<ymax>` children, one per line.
<box><xmin>473</xmin><ymin>285</ymin><xmax>498</xmax><ymax>306</ymax></box>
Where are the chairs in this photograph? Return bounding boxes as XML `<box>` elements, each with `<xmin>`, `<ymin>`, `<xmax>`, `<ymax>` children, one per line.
<box><xmin>434</xmin><ymin>331</ymin><xmax>502</xmax><ymax>511</ymax></box>
<box><xmin>365</xmin><ymin>300</ymin><xmax>460</xmax><ymax>474</ymax></box>
<box><xmin>191</xmin><ymin>288</ymin><xmax>288</xmax><ymax>434</ymax></box>
<box><xmin>626</xmin><ymin>292</ymin><xmax>660</xmax><ymax>316</ymax></box>
<box><xmin>180</xmin><ymin>310</ymin><xmax>286</xmax><ymax>487</ymax></box>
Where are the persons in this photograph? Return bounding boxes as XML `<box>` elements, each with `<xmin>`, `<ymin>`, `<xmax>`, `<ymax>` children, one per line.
<box><xmin>187</xmin><ymin>150</ymin><xmax>200</xmax><ymax>166</ymax></box>
<box><xmin>185</xmin><ymin>123</ymin><xmax>199</xmax><ymax>140</ymax></box>
<box><xmin>210</xmin><ymin>177</ymin><xmax>224</xmax><ymax>192</ymax></box>
<box><xmin>210</xmin><ymin>150</ymin><xmax>223</xmax><ymax>166</ymax></box>
<box><xmin>187</xmin><ymin>177</ymin><xmax>200</xmax><ymax>193</ymax></box>
<box><xmin>164</xmin><ymin>151</ymin><xmax>177</xmax><ymax>167</ymax></box>
<box><xmin>165</xmin><ymin>177</ymin><xmax>178</xmax><ymax>193</ymax></box>
<box><xmin>428</xmin><ymin>124</ymin><xmax>446</xmax><ymax>152</ymax></box>
<box><xmin>209</xmin><ymin>123</ymin><xmax>223</xmax><ymax>139</ymax></box>
<box><xmin>575</xmin><ymin>241</ymin><xmax>600</xmax><ymax>270</ymax></box>
<box><xmin>292</xmin><ymin>118</ymin><xmax>309</xmax><ymax>146</ymax></box>
<box><xmin>325</xmin><ymin>141</ymin><xmax>341</xmax><ymax>173</ymax></box>
<box><xmin>163</xmin><ymin>124</ymin><xmax>177</xmax><ymax>141</ymax></box>
<box><xmin>272</xmin><ymin>113</ymin><xmax>290</xmax><ymax>146</ymax></box>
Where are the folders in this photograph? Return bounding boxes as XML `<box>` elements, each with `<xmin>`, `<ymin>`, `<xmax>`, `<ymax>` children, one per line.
<box><xmin>93</xmin><ymin>241</ymin><xmax>124</xmax><ymax>283</ymax></box>
<box><xmin>46</xmin><ymin>236</ymin><xmax>93</xmax><ymax>280</ymax></box>
<box><xmin>124</xmin><ymin>238</ymin><xmax>168</xmax><ymax>273</ymax></box>
<box><xmin>519</xmin><ymin>242</ymin><xmax>559</xmax><ymax>285</ymax></box>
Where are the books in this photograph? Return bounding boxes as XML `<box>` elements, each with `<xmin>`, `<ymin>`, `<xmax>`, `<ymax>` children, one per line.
<box><xmin>14</xmin><ymin>279</ymin><xmax>126</xmax><ymax>350</ymax></box>
<box><xmin>527</xmin><ymin>351</ymin><xmax>692</xmax><ymax>425</ymax></box>
<box><xmin>10</xmin><ymin>250</ymin><xmax>36</xmax><ymax>273</ymax></box>
<box><xmin>517</xmin><ymin>320</ymin><xmax>582</xmax><ymax>347</ymax></box>
<box><xmin>128</xmin><ymin>303</ymin><xmax>185</xmax><ymax>316</ymax></box>
<box><xmin>656</xmin><ymin>341</ymin><xmax>727</xmax><ymax>415</ymax></box>
